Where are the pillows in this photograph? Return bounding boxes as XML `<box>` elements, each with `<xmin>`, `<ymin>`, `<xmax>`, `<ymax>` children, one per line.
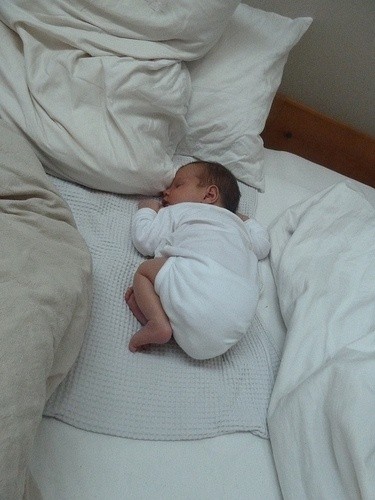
<box><xmin>2</xmin><ymin>0</ymin><xmax>242</xmax><ymax>194</ymax></box>
<box><xmin>177</xmin><ymin>3</ymin><xmax>321</xmax><ymax>188</ymax></box>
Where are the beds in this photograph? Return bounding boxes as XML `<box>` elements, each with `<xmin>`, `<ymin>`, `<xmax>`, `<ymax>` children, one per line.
<box><xmin>2</xmin><ymin>84</ymin><xmax>375</xmax><ymax>500</ymax></box>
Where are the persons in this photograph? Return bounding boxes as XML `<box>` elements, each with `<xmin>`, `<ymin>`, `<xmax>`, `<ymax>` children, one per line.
<box><xmin>124</xmin><ymin>160</ymin><xmax>260</xmax><ymax>361</ymax></box>
